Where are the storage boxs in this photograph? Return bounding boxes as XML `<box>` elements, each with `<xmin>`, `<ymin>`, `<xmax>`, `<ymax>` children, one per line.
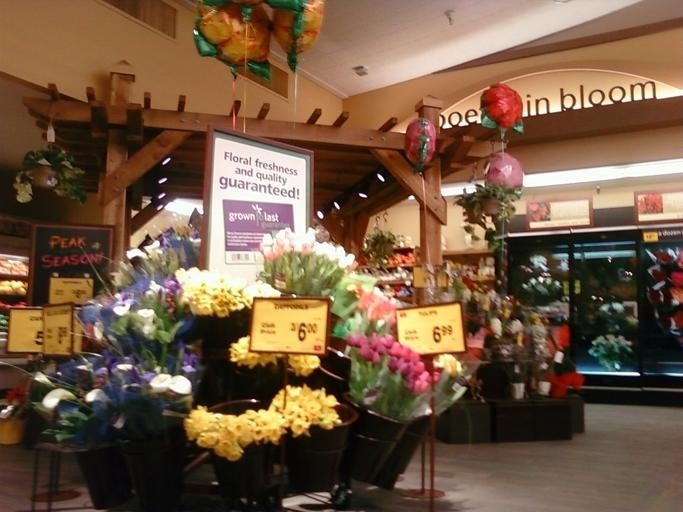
<box><xmin>436</xmin><ymin>400</ymin><xmax>493</xmax><ymax>443</ymax></box>
<box><xmin>494</xmin><ymin>394</ymin><xmax>571</xmax><ymax>441</ymax></box>
<box><xmin>566</xmin><ymin>392</ymin><xmax>585</xmax><ymax>432</ymax></box>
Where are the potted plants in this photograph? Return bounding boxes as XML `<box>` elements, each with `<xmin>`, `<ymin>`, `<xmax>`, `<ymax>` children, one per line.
<box><xmin>13</xmin><ymin>125</ymin><xmax>85</xmax><ymax>204</ymax></box>
<box><xmin>456</xmin><ymin>180</ymin><xmax>523</xmax><ymax>250</ymax></box>
<box><xmin>365</xmin><ymin>210</ymin><xmax>397</xmax><ymax>263</ymax></box>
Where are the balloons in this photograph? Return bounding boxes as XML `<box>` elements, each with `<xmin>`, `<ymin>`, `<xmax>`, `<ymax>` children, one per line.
<box><xmin>193</xmin><ymin>1</ymin><xmax>273</xmax><ymax>83</ymax></box>
<box><xmin>272</xmin><ymin>1</ymin><xmax>325</xmax><ymax>74</ymax></box>
<box><xmin>484</xmin><ymin>152</ymin><xmax>522</xmax><ymax>198</ymax></box>
<box><xmin>405</xmin><ymin>117</ymin><xmax>435</xmax><ymax>170</ymax></box>
<box><xmin>480</xmin><ymin>83</ymin><xmax>524</xmax><ymax>141</ymax></box>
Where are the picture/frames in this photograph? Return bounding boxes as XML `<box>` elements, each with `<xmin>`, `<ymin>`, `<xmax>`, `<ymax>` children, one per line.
<box><xmin>200</xmin><ymin>122</ymin><xmax>314</xmax><ymax>292</ymax></box>
<box><xmin>30</xmin><ymin>221</ymin><xmax>118</xmax><ymax>307</ymax></box>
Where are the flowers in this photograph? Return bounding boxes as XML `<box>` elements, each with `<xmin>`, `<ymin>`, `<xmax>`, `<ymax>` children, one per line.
<box><xmin>437</xmin><ymin>279</ymin><xmax>565</xmax><ymax>398</ymax></box>
<box><xmin>30</xmin><ymin>222</ymin><xmax>468</xmax><ymax>507</ymax></box>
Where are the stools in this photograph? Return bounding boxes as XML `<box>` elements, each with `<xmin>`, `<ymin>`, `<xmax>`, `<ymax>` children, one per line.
<box><xmin>32</xmin><ymin>438</ymin><xmax>84</xmax><ymax>510</ymax></box>
